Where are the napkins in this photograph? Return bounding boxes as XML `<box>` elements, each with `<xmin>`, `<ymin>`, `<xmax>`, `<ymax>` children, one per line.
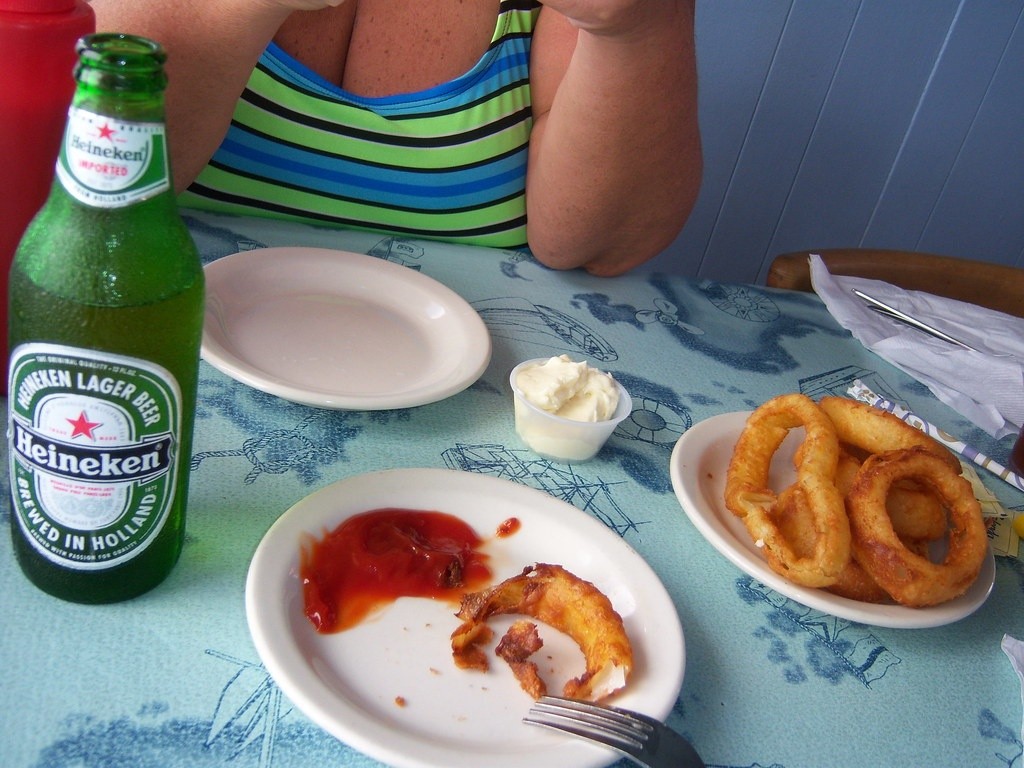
<box><xmin>807</xmin><ymin>252</ymin><xmax>1024</xmax><ymax>452</ymax></box>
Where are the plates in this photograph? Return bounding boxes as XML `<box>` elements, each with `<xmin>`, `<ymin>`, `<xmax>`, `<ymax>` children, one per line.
<box><xmin>242</xmin><ymin>467</ymin><xmax>688</xmax><ymax>768</ymax></box>
<box><xmin>668</xmin><ymin>409</ymin><xmax>996</xmax><ymax>630</ymax></box>
<box><xmin>187</xmin><ymin>246</ymin><xmax>493</xmax><ymax>413</ymax></box>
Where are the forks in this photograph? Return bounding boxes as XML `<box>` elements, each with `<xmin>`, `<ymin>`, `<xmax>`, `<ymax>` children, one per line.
<box><xmin>522</xmin><ymin>695</ymin><xmax>709</xmax><ymax>768</ymax></box>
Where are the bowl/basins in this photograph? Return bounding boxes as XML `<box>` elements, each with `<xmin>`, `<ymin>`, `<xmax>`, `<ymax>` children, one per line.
<box><xmin>508</xmin><ymin>356</ymin><xmax>634</xmax><ymax>462</ymax></box>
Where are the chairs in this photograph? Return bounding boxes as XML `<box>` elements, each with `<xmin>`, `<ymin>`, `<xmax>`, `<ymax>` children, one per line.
<box><xmin>765</xmin><ymin>249</ymin><xmax>1024</xmax><ymax>319</ymax></box>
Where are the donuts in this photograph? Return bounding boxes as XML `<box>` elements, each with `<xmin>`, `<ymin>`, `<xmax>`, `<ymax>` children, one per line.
<box><xmin>720</xmin><ymin>395</ymin><xmax>988</xmax><ymax>609</ymax></box>
<box><xmin>450</xmin><ymin>561</ymin><xmax>632</xmax><ymax>704</ymax></box>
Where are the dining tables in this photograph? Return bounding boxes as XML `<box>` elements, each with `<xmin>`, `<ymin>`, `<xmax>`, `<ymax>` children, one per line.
<box><xmin>0</xmin><ymin>205</ymin><xmax>1024</xmax><ymax>768</ymax></box>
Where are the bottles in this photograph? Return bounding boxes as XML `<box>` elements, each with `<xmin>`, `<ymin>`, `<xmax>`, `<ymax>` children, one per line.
<box><xmin>5</xmin><ymin>30</ymin><xmax>207</xmax><ymax>606</ymax></box>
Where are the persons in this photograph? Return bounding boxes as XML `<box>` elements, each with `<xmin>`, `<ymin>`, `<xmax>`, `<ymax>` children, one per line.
<box><xmin>84</xmin><ymin>0</ymin><xmax>705</xmax><ymax>275</ymax></box>
